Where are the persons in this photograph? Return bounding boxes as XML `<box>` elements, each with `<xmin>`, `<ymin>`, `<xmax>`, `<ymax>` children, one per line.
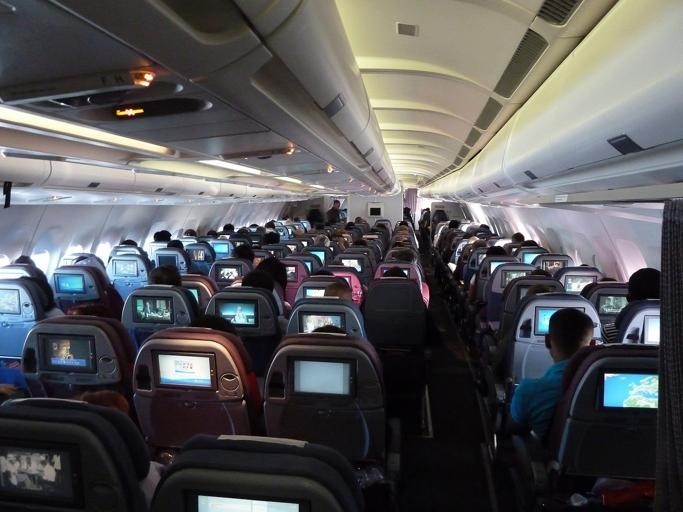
<box><xmin>1</xmin><ymin>200</ymin><xmax>660</xmax><ymax>512</ymax></box>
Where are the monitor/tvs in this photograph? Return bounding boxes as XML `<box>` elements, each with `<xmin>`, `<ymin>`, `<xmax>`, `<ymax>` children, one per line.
<box><xmin>565</xmin><ymin>275</ymin><xmax>597</xmax><ymax>295</ymax></box>
<box><xmin>370</xmin><ymin>207</ymin><xmax>381</xmax><ymax>215</ymax></box>
<box><xmin>277</xmin><ymin>230</ymin><xmax>284</xmax><ymax>235</ymax></box>
<box><xmin>287</xmin><ymin>245</ymin><xmax>298</xmax><ymax>253</ymax></box>
<box><xmin>517</xmin><ymin>286</ymin><xmax>556</xmax><ymax>302</ymax></box>
<box><xmin>285</xmin><ymin>265</ymin><xmax>297</xmax><ymax>283</ymax></box>
<box><xmin>213</xmin><ymin>243</ymin><xmax>229</xmax><ymax>254</ymax></box>
<box><xmin>602</xmin><ymin>370</ymin><xmax>659</xmax><ymax>411</ymax></box>
<box><xmin>252</xmin><ymin>256</ymin><xmax>264</xmax><ymax>269</ymax></box>
<box><xmin>186</xmin><ymin>288</ymin><xmax>200</xmax><ymax>304</ymax></box>
<box><xmin>490</xmin><ymin>261</ymin><xmax>514</xmax><ymax>274</ymax></box>
<box><xmin>112</xmin><ymin>260</ymin><xmax>138</xmax><ymax>277</ymax></box>
<box><xmin>303</xmin><ymin>286</ymin><xmax>325</xmax><ymax>297</ymax></box>
<box><xmin>599</xmin><ymin>294</ymin><xmax>628</xmax><ymax>313</ymax></box>
<box><xmin>53</xmin><ymin>273</ymin><xmax>86</xmax><ymax>294</ymax></box>
<box><xmin>117</xmin><ymin>251</ymin><xmax>135</xmax><ymax>256</ymax></box>
<box><xmin>132</xmin><ymin>295</ymin><xmax>173</xmax><ymax>324</ymax></box>
<box><xmin>644</xmin><ymin>315</ymin><xmax>661</xmax><ymax>344</ymax></box>
<box><xmin>39</xmin><ymin>334</ymin><xmax>96</xmax><ymax>374</ymax></box>
<box><xmin>152</xmin><ymin>351</ymin><xmax>217</xmax><ymax>392</ymax></box>
<box><xmin>478</xmin><ymin>253</ymin><xmax>487</xmax><ymax>266</ymax></box>
<box><xmin>288</xmin><ymin>228</ymin><xmax>291</xmax><ymax>234</ymax></box>
<box><xmin>0</xmin><ymin>290</ymin><xmax>21</xmax><ymax>315</ymax></box>
<box><xmin>157</xmin><ymin>255</ymin><xmax>177</xmax><ymax>268</ymax></box>
<box><xmin>298</xmin><ymin>311</ymin><xmax>346</xmax><ymax>333</ymax></box>
<box><xmin>0</xmin><ymin>445</ymin><xmax>77</xmax><ymax>504</ymax></box>
<box><xmin>216</xmin><ymin>299</ymin><xmax>258</xmax><ymax>327</ymax></box>
<box><xmin>339</xmin><ymin>258</ymin><xmax>363</xmax><ymax>272</ymax></box>
<box><xmin>288</xmin><ymin>357</ymin><xmax>357</xmax><ymax>397</ymax></box>
<box><xmin>216</xmin><ymin>265</ymin><xmax>242</xmax><ymax>282</ymax></box>
<box><xmin>303</xmin><ymin>261</ymin><xmax>313</xmax><ymax>274</ymax></box>
<box><xmin>310</xmin><ymin>252</ymin><xmax>325</xmax><ymax>265</ymax></box>
<box><xmin>250</xmin><ymin>235</ymin><xmax>261</xmax><ymax>242</ymax></box>
<box><xmin>383</xmin><ymin>269</ymin><xmax>410</xmax><ymax>277</ymax></box>
<box><xmin>330</xmin><ymin>246</ymin><xmax>334</xmax><ymax>251</ymax></box>
<box><xmin>534</xmin><ymin>306</ymin><xmax>585</xmax><ymax>336</ymax></box>
<box><xmin>234</xmin><ymin>241</ymin><xmax>244</xmax><ymax>247</ymax></box>
<box><xmin>185</xmin><ymin>249</ymin><xmax>205</xmax><ymax>261</ymax></box>
<box><xmin>342</xmin><ymin>276</ymin><xmax>351</xmax><ymax>284</ymax></box>
<box><xmin>301</xmin><ymin>241</ymin><xmax>308</xmax><ymax>247</ymax></box>
<box><xmin>295</xmin><ymin>225</ymin><xmax>299</xmax><ymax>229</ymax></box>
<box><xmin>542</xmin><ymin>260</ymin><xmax>568</xmax><ymax>272</ymax></box>
<box><xmin>523</xmin><ymin>253</ymin><xmax>544</xmax><ymax>264</ymax></box>
<box><xmin>501</xmin><ymin>270</ymin><xmax>531</xmax><ymax>288</ymax></box>
<box><xmin>198</xmin><ymin>491</ymin><xmax>301</xmax><ymax>512</ymax></box>
<box><xmin>303</xmin><ymin>223</ymin><xmax>307</xmax><ymax>227</ymax></box>
<box><xmin>510</xmin><ymin>246</ymin><xmax>520</xmax><ymax>253</ymax></box>
